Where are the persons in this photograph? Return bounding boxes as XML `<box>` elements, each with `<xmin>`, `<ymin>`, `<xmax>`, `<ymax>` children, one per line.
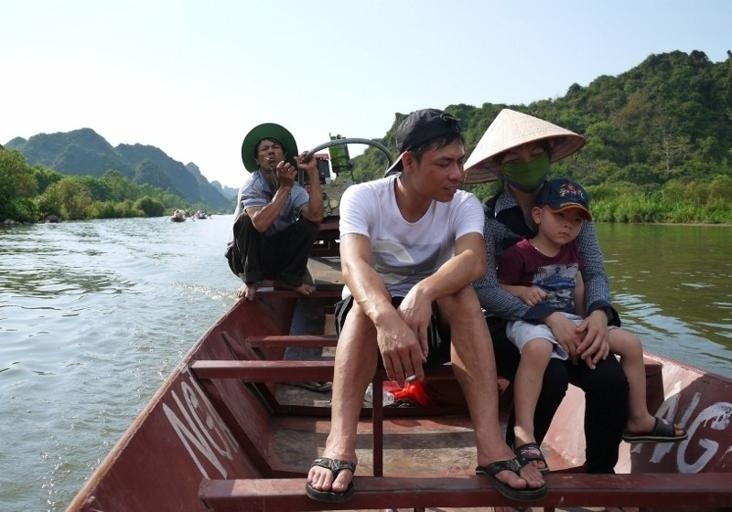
<box><xmin>224</xmin><ymin>122</ymin><xmax>324</xmax><ymax>302</ymax></box>
<box><xmin>498</xmin><ymin>179</ymin><xmax>688</xmax><ymax>473</ymax></box>
<box><xmin>460</xmin><ymin>108</ymin><xmax>629</xmax><ymax>474</ymax></box>
<box><xmin>306</xmin><ymin>107</ymin><xmax>547</xmax><ymax>503</ymax></box>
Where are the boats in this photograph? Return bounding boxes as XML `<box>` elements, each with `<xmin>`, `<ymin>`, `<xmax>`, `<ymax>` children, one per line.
<box><xmin>170</xmin><ymin>215</ymin><xmax>186</xmax><ymax>222</ymax></box>
<box><xmin>195</xmin><ymin>214</ymin><xmax>207</xmax><ymax>219</ymax></box>
<box><xmin>64</xmin><ymin>136</ymin><xmax>732</xmax><ymax>512</ymax></box>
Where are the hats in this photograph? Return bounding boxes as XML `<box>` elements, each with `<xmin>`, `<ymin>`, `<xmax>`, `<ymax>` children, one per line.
<box><xmin>535</xmin><ymin>179</ymin><xmax>593</xmax><ymax>222</ymax></box>
<box><xmin>242</xmin><ymin>123</ymin><xmax>298</xmax><ymax>173</ymax></box>
<box><xmin>462</xmin><ymin>108</ymin><xmax>586</xmax><ymax>185</ymax></box>
<box><xmin>384</xmin><ymin>108</ymin><xmax>461</xmax><ymax>178</ymax></box>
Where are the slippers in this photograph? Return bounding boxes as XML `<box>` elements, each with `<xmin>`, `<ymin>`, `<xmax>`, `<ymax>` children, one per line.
<box><xmin>305</xmin><ymin>458</ymin><xmax>356</xmax><ymax>504</ymax></box>
<box><xmin>475</xmin><ymin>456</ymin><xmax>547</xmax><ymax>503</ymax></box>
<box><xmin>512</xmin><ymin>441</ymin><xmax>550</xmax><ymax>476</ymax></box>
<box><xmin>624</xmin><ymin>416</ymin><xmax>688</xmax><ymax>442</ymax></box>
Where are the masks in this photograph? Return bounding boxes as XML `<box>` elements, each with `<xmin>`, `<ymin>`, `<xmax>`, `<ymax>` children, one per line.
<box><xmin>499</xmin><ymin>151</ymin><xmax>549</xmax><ymax>188</ymax></box>
